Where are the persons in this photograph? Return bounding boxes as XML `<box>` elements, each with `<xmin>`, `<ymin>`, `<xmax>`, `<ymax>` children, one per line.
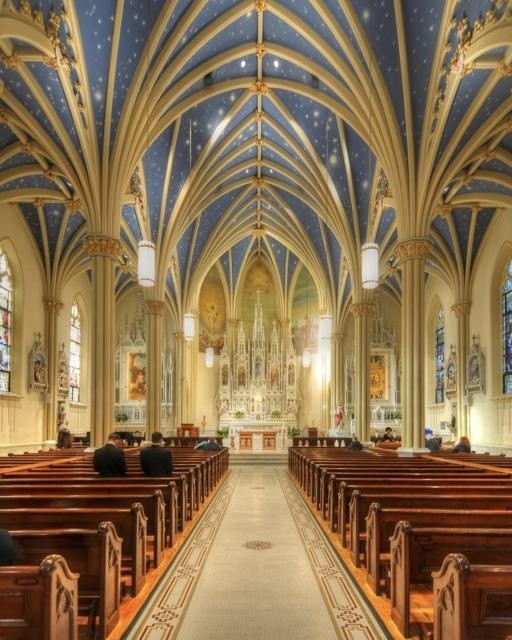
<box><xmin>383</xmin><ymin>427</ymin><xmax>395</xmax><ymax>440</ymax></box>
<box><xmin>140</xmin><ymin>432</ymin><xmax>172</xmax><ymax>477</ymax></box>
<box><xmin>426</xmin><ymin>435</ymin><xmax>444</xmax><ymax>450</ymax></box>
<box><xmin>202</xmin><ymin>436</ymin><xmax>221</xmax><ymax>450</ymax></box>
<box><xmin>193</xmin><ymin>439</ymin><xmax>208</xmax><ymax>449</ymax></box>
<box><xmin>93</xmin><ymin>432</ymin><xmax>129</xmax><ymax>478</ymax></box>
<box><xmin>451</xmin><ymin>435</ymin><xmax>472</xmax><ymax>453</ymax></box>
<box><xmin>347</xmin><ymin>438</ymin><xmax>365</xmax><ymax>451</ymax></box>
<box><xmin>55</xmin><ymin>423</ymin><xmax>74</xmax><ymax>449</ymax></box>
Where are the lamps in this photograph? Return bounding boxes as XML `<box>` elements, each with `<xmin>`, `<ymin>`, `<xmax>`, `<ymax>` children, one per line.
<box><xmin>301</xmin><ymin>266</ymin><xmax>311</xmax><ymax>368</ymax></box>
<box><xmin>319</xmin><ymin>110</ymin><xmax>332</xmax><ymax>339</ymax></box>
<box><xmin>137</xmin><ymin>1</ymin><xmax>157</xmax><ymax>287</ymax></box>
<box><xmin>360</xmin><ymin>0</ymin><xmax>380</xmax><ymax>289</ymax></box>
<box><xmin>183</xmin><ymin>120</ymin><xmax>195</xmax><ymax>342</ymax></box>
<box><xmin>205</xmin><ymin>268</ymin><xmax>214</xmax><ymax>367</ymax></box>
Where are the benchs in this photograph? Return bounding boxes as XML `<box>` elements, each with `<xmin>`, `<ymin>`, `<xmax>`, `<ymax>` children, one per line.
<box><xmin>1</xmin><ymin>446</ymin><xmax>231</xmax><ymax>640</ymax></box>
<box><xmin>287</xmin><ymin>446</ymin><xmax>512</xmax><ymax>639</ymax></box>
